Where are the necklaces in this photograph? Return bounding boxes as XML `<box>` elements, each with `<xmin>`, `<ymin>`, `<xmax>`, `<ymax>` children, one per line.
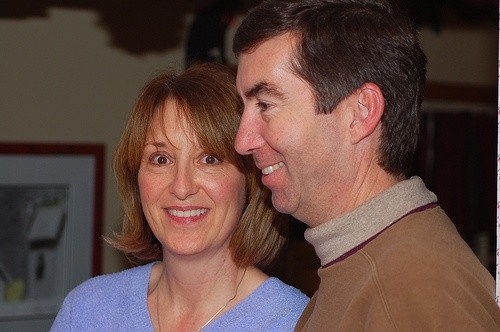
<box><xmin>157</xmin><ymin>262</ymin><xmax>249</xmax><ymax>332</ymax></box>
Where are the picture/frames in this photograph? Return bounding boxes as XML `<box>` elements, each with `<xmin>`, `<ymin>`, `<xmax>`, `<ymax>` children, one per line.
<box><xmin>0</xmin><ymin>142</ymin><xmax>107</xmax><ymax>331</ymax></box>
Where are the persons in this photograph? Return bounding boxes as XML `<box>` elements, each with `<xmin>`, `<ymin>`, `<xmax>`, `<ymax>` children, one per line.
<box><xmin>49</xmin><ymin>63</ymin><xmax>313</xmax><ymax>332</ymax></box>
<box><xmin>231</xmin><ymin>0</ymin><xmax>500</xmax><ymax>331</ymax></box>
